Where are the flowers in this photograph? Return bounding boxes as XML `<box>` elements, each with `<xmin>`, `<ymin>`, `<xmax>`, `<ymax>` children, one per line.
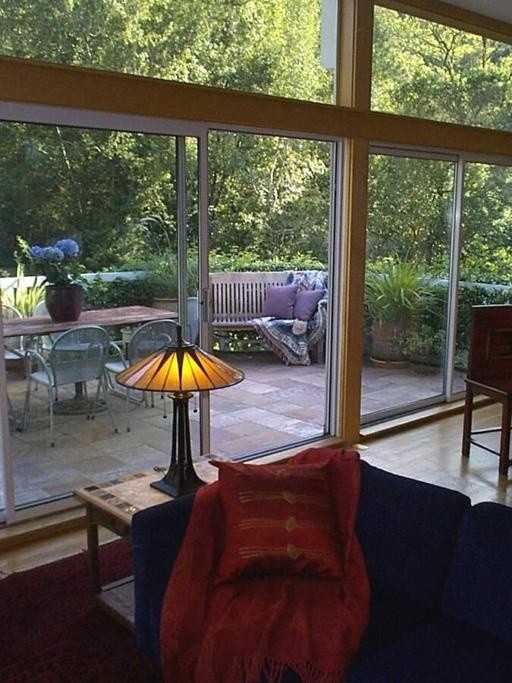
<box><xmin>29</xmin><ymin>238</ymin><xmax>80</xmax><ymax>287</ymax></box>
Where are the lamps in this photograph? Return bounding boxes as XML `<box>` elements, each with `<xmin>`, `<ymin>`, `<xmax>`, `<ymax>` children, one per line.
<box><xmin>113</xmin><ymin>324</ymin><xmax>247</xmax><ymax>498</ymax></box>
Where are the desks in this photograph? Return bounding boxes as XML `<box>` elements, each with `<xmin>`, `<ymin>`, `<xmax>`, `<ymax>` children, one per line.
<box><xmin>70</xmin><ymin>450</ymin><xmax>237</xmax><ymax>643</ymax></box>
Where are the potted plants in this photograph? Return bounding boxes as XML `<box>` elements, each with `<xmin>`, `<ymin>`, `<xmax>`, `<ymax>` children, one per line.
<box><xmin>364</xmin><ymin>248</ymin><xmax>446</xmax><ymax>369</ymax></box>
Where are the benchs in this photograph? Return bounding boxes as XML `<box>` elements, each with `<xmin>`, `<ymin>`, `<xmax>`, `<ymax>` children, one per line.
<box><xmin>207</xmin><ymin>269</ymin><xmax>328</xmax><ymax>366</ymax></box>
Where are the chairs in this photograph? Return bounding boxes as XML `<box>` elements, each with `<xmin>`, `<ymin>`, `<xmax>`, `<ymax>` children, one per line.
<box><xmin>0</xmin><ymin>295</ymin><xmax>199</xmax><ymax>446</ymax></box>
<box><xmin>462</xmin><ymin>303</ymin><xmax>512</xmax><ymax>476</ymax></box>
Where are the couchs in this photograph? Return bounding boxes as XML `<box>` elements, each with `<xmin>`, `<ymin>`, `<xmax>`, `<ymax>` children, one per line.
<box><xmin>129</xmin><ymin>455</ymin><xmax>512</xmax><ymax>680</ymax></box>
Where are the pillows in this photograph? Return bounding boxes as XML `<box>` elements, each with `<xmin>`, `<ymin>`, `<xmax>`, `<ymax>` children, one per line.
<box><xmin>207</xmin><ymin>456</ymin><xmax>346</xmax><ymax>587</ymax></box>
<box><xmin>291</xmin><ymin>288</ymin><xmax>326</xmax><ymax>321</ymax></box>
<box><xmin>262</xmin><ymin>284</ymin><xmax>299</xmax><ymax>318</ymax></box>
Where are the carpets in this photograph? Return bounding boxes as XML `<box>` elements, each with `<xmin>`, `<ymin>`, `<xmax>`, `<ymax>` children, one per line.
<box><xmin>0</xmin><ymin>535</ymin><xmax>157</xmax><ymax>681</ymax></box>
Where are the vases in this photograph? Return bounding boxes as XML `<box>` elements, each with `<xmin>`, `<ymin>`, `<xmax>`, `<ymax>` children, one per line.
<box><xmin>42</xmin><ymin>284</ymin><xmax>84</xmax><ymax>322</ymax></box>
